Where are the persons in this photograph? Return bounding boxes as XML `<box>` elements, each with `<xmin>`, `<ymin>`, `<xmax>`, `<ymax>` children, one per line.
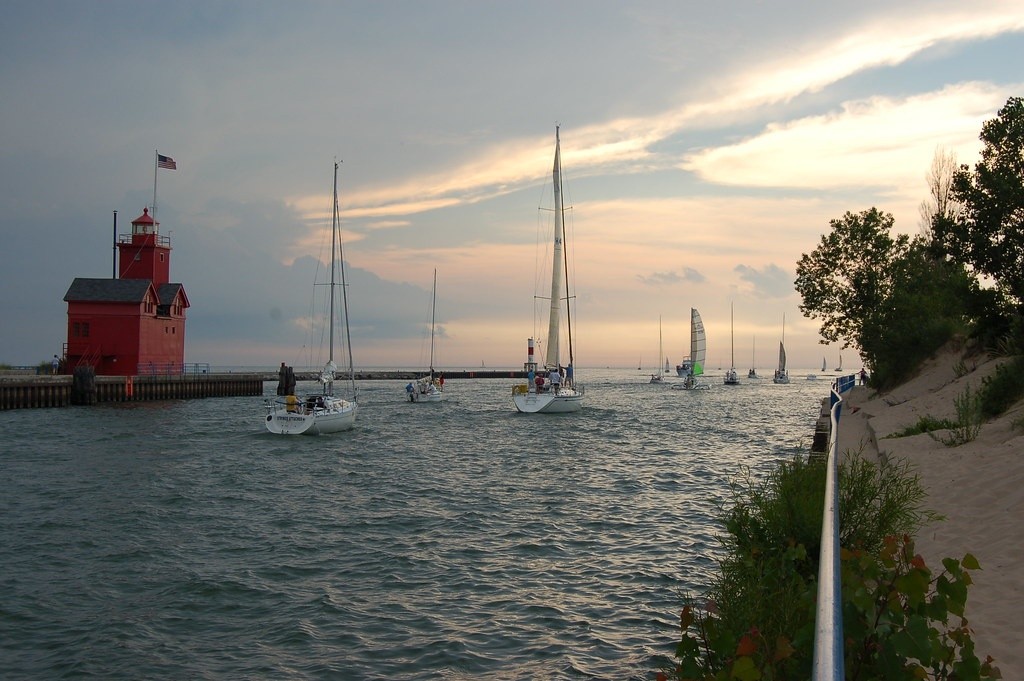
<box><xmin>528</xmin><ymin>364</ymin><xmax>573</xmax><ymax>394</ymax></box>
<box><xmin>52</xmin><ymin>355</ymin><xmax>59</xmax><ymax>376</ymax></box>
<box><xmin>316</xmin><ymin>397</ymin><xmax>328</xmax><ymax>410</ymax></box>
<box><xmin>856</xmin><ymin>368</ymin><xmax>867</xmax><ymax>386</ymax></box>
<box><xmin>286</xmin><ymin>388</ymin><xmax>301</xmax><ymax>415</ymax></box>
<box><xmin>406</xmin><ymin>369</ymin><xmax>445</xmax><ymax>397</ymax></box>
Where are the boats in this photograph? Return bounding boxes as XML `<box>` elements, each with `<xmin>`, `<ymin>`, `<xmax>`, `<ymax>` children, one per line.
<box><xmin>806</xmin><ymin>374</ymin><xmax>817</xmax><ymax>381</ymax></box>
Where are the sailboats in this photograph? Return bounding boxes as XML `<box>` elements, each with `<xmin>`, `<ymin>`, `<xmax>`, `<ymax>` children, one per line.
<box><xmin>260</xmin><ymin>161</ymin><xmax>360</xmax><ymax>436</ymax></box>
<box><xmin>773</xmin><ymin>340</ymin><xmax>790</xmax><ymax>384</ymax></box>
<box><xmin>669</xmin><ymin>307</ymin><xmax>711</xmax><ymax>390</ymax></box>
<box><xmin>820</xmin><ymin>356</ymin><xmax>827</xmax><ymax>372</ymax></box>
<box><xmin>723</xmin><ymin>299</ymin><xmax>741</xmax><ymax>385</ymax></box>
<box><xmin>649</xmin><ymin>313</ymin><xmax>671</xmax><ymax>385</ymax></box>
<box><xmin>747</xmin><ymin>334</ymin><xmax>759</xmax><ymax>378</ymax></box>
<box><xmin>511</xmin><ymin>125</ymin><xmax>587</xmax><ymax>414</ymax></box>
<box><xmin>406</xmin><ymin>268</ymin><xmax>443</xmax><ymax>403</ymax></box>
<box><xmin>835</xmin><ymin>355</ymin><xmax>843</xmax><ymax>371</ymax></box>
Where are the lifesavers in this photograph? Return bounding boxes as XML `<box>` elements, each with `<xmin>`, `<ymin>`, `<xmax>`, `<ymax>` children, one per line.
<box><xmin>512</xmin><ymin>385</ymin><xmax>523</xmax><ymax>395</ymax></box>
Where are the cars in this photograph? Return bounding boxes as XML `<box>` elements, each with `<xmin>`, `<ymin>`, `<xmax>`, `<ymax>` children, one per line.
<box><xmin>676</xmin><ymin>353</ymin><xmax>691</xmax><ymax>379</ymax></box>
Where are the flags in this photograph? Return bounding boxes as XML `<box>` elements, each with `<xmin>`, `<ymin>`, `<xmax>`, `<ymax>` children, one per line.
<box><xmin>157</xmin><ymin>153</ymin><xmax>177</xmax><ymax>170</ymax></box>
